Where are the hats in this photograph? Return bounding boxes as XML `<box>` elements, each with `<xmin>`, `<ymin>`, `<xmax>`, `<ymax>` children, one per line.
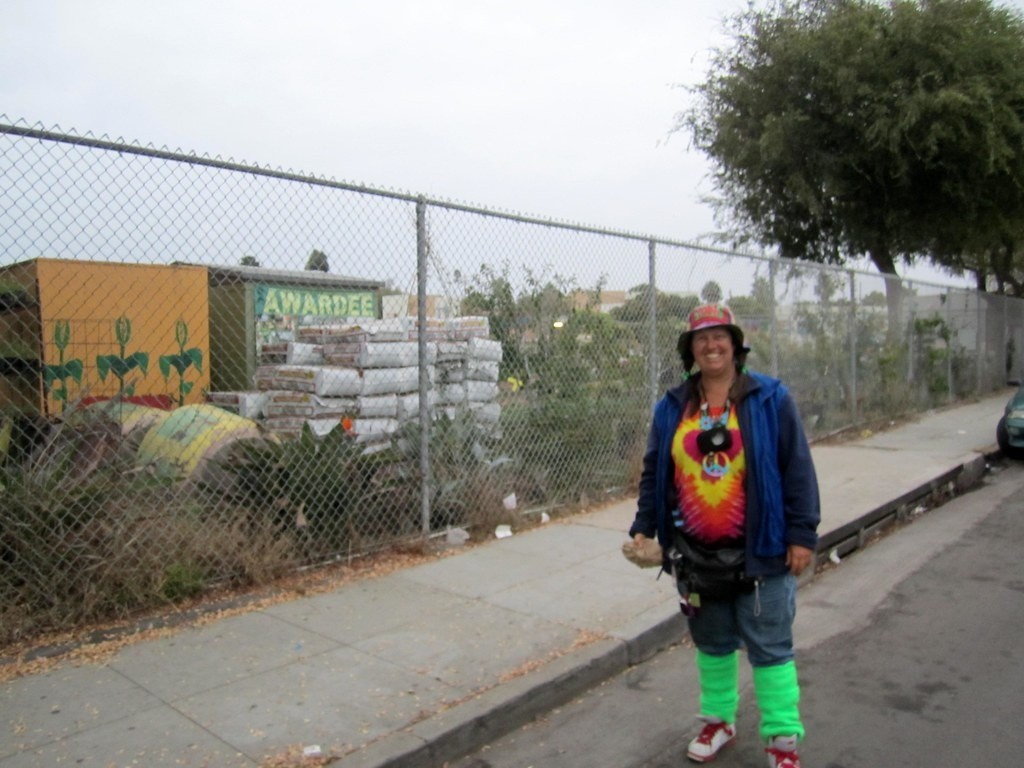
<box><xmin>678</xmin><ymin>303</ymin><xmax>744</xmax><ymax>354</ymax></box>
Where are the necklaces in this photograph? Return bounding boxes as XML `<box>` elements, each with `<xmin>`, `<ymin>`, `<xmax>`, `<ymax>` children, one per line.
<box><xmin>697</xmin><ymin>381</ymin><xmax>733</xmax><ymax>476</ymax></box>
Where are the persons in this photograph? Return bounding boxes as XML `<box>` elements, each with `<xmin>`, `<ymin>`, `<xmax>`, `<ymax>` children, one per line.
<box><xmin>627</xmin><ymin>302</ymin><xmax>821</xmax><ymax>768</ymax></box>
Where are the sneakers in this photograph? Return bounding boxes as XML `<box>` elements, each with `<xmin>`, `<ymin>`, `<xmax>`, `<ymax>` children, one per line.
<box><xmin>687</xmin><ymin>714</ymin><xmax>736</xmax><ymax>762</ymax></box>
<box><xmin>764</xmin><ymin>733</ymin><xmax>800</xmax><ymax>768</ymax></box>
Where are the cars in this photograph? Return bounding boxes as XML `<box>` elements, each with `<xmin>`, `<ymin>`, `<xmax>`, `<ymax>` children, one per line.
<box><xmin>996</xmin><ymin>380</ymin><xmax>1024</xmax><ymax>460</ymax></box>
